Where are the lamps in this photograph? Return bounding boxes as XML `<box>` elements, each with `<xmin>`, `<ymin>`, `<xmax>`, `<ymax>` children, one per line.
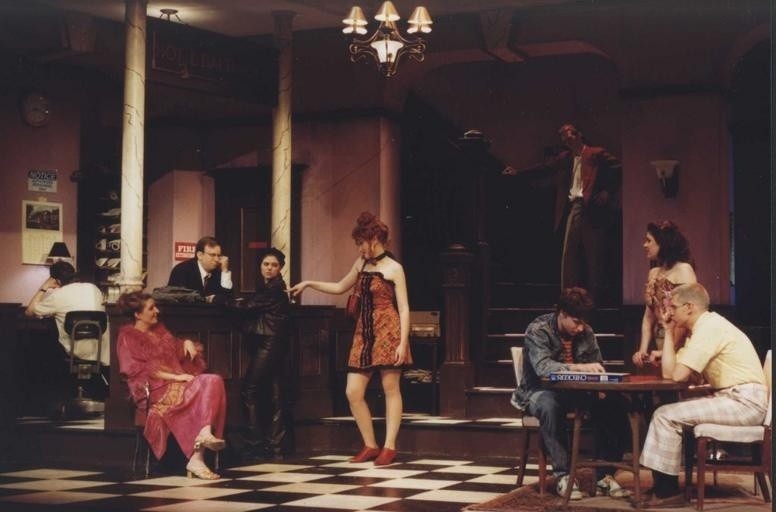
<box><xmin>650</xmin><ymin>159</ymin><xmax>680</xmax><ymax>199</ymax></box>
<box><xmin>341</xmin><ymin>0</ymin><xmax>434</xmax><ymax>81</ymax></box>
<box><xmin>48</xmin><ymin>242</ymin><xmax>71</xmax><ymax>262</ymax></box>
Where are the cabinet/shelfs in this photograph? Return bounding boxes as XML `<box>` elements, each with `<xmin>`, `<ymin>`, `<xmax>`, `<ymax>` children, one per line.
<box><xmin>93</xmin><ymin>200</ymin><xmax>148</xmax><ymax>287</ymax></box>
<box><xmin>202</xmin><ymin>163</ymin><xmax>311</xmax><ymax>305</ymax></box>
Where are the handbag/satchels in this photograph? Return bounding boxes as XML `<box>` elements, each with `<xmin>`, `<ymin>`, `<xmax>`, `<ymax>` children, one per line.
<box><xmin>345</xmin><ymin>294</ymin><xmax>361</xmax><ymax>321</ymax></box>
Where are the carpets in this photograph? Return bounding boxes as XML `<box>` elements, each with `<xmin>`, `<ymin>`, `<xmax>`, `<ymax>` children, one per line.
<box><xmin>460</xmin><ymin>469</ymin><xmax>772</xmax><ymax>512</ymax></box>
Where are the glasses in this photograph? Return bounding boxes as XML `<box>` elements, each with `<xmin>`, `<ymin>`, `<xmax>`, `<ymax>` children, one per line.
<box><xmin>205</xmin><ymin>252</ymin><xmax>223</xmax><ymax>259</ymax></box>
<box><xmin>671</xmin><ymin>302</ymin><xmax>689</xmax><ymax>311</ymax></box>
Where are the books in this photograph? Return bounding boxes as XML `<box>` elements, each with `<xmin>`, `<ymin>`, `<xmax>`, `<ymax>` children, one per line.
<box><xmin>550</xmin><ymin>371</ymin><xmax>631</xmax><ymax>383</ymax></box>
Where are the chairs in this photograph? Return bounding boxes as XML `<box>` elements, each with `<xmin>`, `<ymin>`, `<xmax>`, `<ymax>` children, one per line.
<box><xmin>119</xmin><ymin>375</ymin><xmax>220</xmax><ymax>479</ymax></box>
<box><xmin>60</xmin><ymin>311</ymin><xmax>109</xmax><ymax>419</ymax></box>
<box><xmin>510</xmin><ymin>346</ymin><xmax>592</xmax><ymax>503</ymax></box>
<box><xmin>684</xmin><ymin>349</ymin><xmax>772</xmax><ymax>512</ymax></box>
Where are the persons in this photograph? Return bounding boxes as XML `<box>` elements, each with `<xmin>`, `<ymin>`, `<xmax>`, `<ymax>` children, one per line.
<box><xmin>509</xmin><ymin>286</ymin><xmax>634</xmax><ymax>502</ymax></box>
<box><xmin>208</xmin><ymin>246</ymin><xmax>292</xmax><ymax>461</ymax></box>
<box><xmin>25</xmin><ymin>261</ymin><xmax>110</xmax><ymax>379</ymax></box>
<box><xmin>282</xmin><ymin>210</ymin><xmax>413</xmax><ymax>465</ymax></box>
<box><xmin>629</xmin><ymin>283</ymin><xmax>769</xmax><ymax>509</ymax></box>
<box><xmin>169</xmin><ymin>238</ymin><xmax>234</xmax><ymax>305</ymax></box>
<box><xmin>116</xmin><ymin>292</ymin><xmax>227</xmax><ymax>479</ymax></box>
<box><xmin>631</xmin><ymin>222</ymin><xmax>698</xmax><ymax>368</ymax></box>
<box><xmin>500</xmin><ymin>122</ymin><xmax>618</xmax><ymax>308</ymax></box>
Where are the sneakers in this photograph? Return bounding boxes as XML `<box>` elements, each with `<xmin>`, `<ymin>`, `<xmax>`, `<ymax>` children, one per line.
<box><xmin>596</xmin><ymin>477</ymin><xmax>633</xmax><ymax>497</ymax></box>
<box><xmin>556</xmin><ymin>475</ymin><xmax>582</xmax><ymax>499</ymax></box>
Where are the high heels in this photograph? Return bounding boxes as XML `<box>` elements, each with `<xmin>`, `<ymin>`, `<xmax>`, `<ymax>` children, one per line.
<box><xmin>186</xmin><ymin>462</ymin><xmax>221</xmax><ymax>480</ymax></box>
<box><xmin>194</xmin><ymin>435</ymin><xmax>227</xmax><ymax>452</ymax></box>
<box><xmin>374</xmin><ymin>448</ymin><xmax>397</xmax><ymax>465</ymax></box>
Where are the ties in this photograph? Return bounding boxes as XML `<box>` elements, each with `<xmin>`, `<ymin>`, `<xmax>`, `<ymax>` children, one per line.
<box><xmin>204</xmin><ymin>275</ymin><xmax>210</xmax><ymax>290</ymax></box>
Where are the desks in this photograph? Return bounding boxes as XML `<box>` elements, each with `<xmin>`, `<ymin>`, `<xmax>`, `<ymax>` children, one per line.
<box><xmin>0</xmin><ymin>303</ymin><xmax>55</xmax><ymax>331</ymax></box>
<box><xmin>101</xmin><ymin>307</ymin><xmax>335</xmax><ymax>431</ymax></box>
<box><xmin>540</xmin><ymin>377</ymin><xmax>682</xmax><ymax>512</ymax></box>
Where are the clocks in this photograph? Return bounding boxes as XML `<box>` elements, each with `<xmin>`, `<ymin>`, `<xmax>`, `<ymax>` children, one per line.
<box><xmin>20</xmin><ymin>93</ymin><xmax>50</xmax><ymax>127</ymax></box>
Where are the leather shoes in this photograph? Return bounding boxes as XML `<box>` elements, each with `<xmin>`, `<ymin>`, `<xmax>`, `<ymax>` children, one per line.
<box><xmin>632</xmin><ymin>492</ymin><xmax>687</xmax><ymax>508</ymax></box>
<box><xmin>349</xmin><ymin>447</ymin><xmax>379</xmax><ymax>462</ymax></box>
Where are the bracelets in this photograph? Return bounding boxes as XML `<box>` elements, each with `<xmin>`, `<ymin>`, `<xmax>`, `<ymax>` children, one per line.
<box><xmin>40</xmin><ymin>288</ymin><xmax>47</xmax><ymax>293</ymax></box>
